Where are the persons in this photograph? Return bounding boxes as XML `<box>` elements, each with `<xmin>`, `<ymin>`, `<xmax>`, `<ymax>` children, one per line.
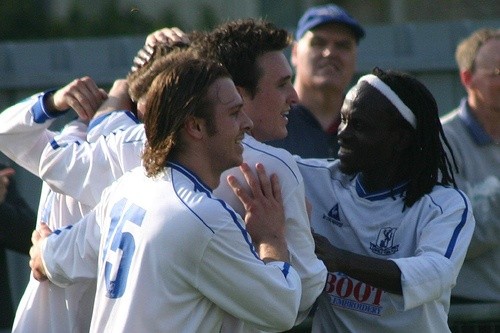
<box><xmin>0</xmin><ymin>163</ymin><xmax>37</xmax><ymax>333</ymax></box>
<box><xmin>28</xmin><ymin>61</ymin><xmax>302</xmax><ymax>333</ymax></box>
<box><xmin>295</xmin><ymin>68</ymin><xmax>475</xmax><ymax>333</ymax></box>
<box><xmin>0</xmin><ymin>18</ymin><xmax>328</xmax><ymax>333</ymax></box>
<box><xmin>435</xmin><ymin>29</ymin><xmax>500</xmax><ymax>333</ymax></box>
<box><xmin>262</xmin><ymin>4</ymin><xmax>366</xmax><ymax>160</ymax></box>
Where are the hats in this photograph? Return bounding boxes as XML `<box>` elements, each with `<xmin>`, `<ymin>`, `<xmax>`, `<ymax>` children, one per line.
<box><xmin>296</xmin><ymin>4</ymin><xmax>366</xmax><ymax>42</ymax></box>
